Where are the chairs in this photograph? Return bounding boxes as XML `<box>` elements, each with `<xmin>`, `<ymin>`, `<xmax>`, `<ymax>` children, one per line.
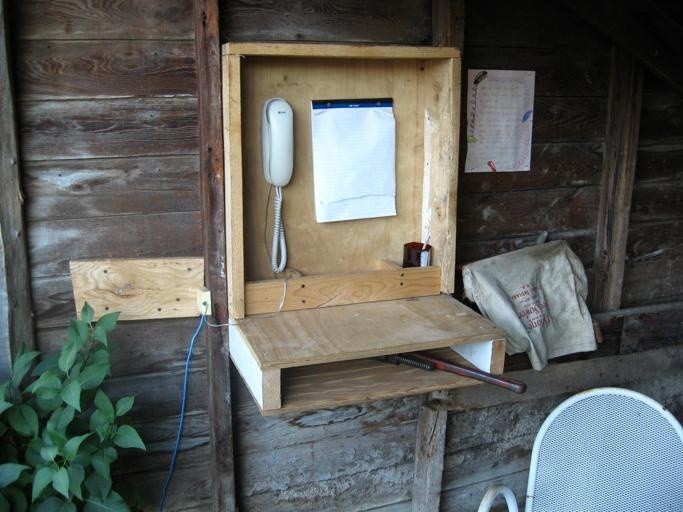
<box><xmin>477</xmin><ymin>387</ymin><xmax>683</xmax><ymax>512</ymax></box>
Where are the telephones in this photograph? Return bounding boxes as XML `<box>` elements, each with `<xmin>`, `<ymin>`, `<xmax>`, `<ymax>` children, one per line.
<box><xmin>262</xmin><ymin>97</ymin><xmax>294</xmax><ymax>192</ymax></box>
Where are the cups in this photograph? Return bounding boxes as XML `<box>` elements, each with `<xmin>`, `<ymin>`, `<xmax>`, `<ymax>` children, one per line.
<box><xmin>403</xmin><ymin>242</ymin><xmax>431</xmax><ymax>267</ymax></box>
